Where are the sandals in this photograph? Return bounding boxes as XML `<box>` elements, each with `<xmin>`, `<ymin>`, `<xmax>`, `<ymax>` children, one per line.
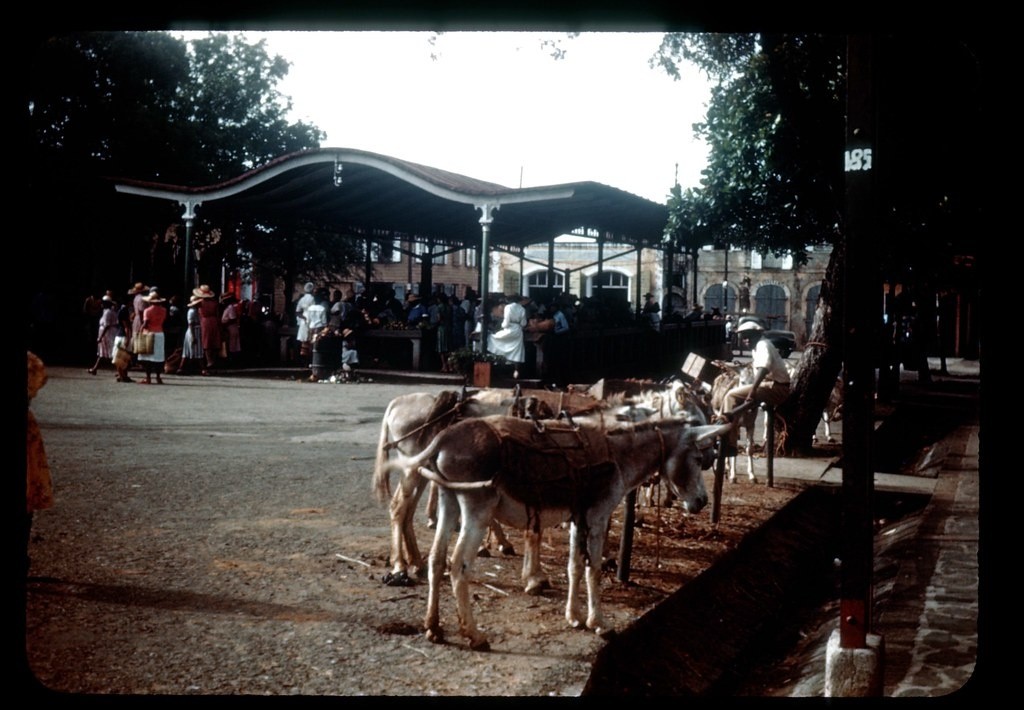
<box><xmin>86</xmin><ymin>369</ymin><xmax>96</xmax><ymax>375</ymax></box>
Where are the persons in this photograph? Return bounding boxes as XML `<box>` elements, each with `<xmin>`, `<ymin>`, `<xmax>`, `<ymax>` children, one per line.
<box><xmin>714</xmin><ymin>322</ymin><xmax>792</xmax><ymax>457</ymax></box>
<box><xmin>27</xmin><ymin>350</ymin><xmax>54</xmax><ymax>569</ymax></box>
<box><xmin>81</xmin><ymin>281</ymin><xmax>733</xmax><ymax>385</ymax></box>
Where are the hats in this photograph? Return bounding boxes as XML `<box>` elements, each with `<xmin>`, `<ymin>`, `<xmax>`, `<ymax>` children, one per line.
<box><xmin>127</xmin><ymin>282</ymin><xmax>149</xmax><ymax>295</ymax></box>
<box><xmin>304</xmin><ymin>282</ymin><xmax>313</xmax><ymax>292</ymax></box>
<box><xmin>643</xmin><ymin>293</ymin><xmax>654</xmax><ymax>297</ymax></box>
<box><xmin>142</xmin><ymin>292</ymin><xmax>166</xmax><ymax>302</ymax></box>
<box><xmin>408</xmin><ymin>294</ymin><xmax>422</xmax><ymax>301</ymax></box>
<box><xmin>187</xmin><ymin>296</ymin><xmax>202</xmax><ymax>307</ymax></box>
<box><xmin>192</xmin><ymin>285</ymin><xmax>215</xmax><ymax>298</ymax></box>
<box><xmin>220</xmin><ymin>292</ymin><xmax>232</xmax><ymax>302</ymax></box>
<box><xmin>725</xmin><ymin>315</ymin><xmax>733</xmax><ymax>320</ymax></box>
<box><xmin>735</xmin><ymin>321</ymin><xmax>764</xmax><ymax>333</ymax></box>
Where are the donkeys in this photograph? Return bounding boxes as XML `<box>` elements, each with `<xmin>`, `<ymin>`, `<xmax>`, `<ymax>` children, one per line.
<box><xmin>371</xmin><ymin>357</ymin><xmax>845</xmax><ymax>652</ymax></box>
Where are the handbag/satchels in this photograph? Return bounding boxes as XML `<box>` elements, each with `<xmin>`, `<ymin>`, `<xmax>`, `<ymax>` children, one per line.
<box><xmin>112</xmin><ymin>346</ymin><xmax>130</xmax><ymax>370</ymax></box>
<box><xmin>133</xmin><ymin>325</ymin><xmax>155</xmax><ymax>354</ymax></box>
